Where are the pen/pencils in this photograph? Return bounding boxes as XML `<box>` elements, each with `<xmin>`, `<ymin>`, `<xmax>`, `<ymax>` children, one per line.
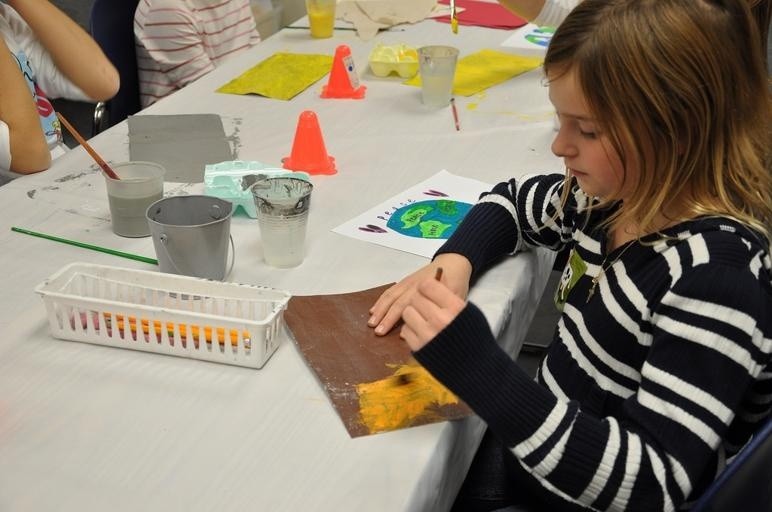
<box><xmin>90</xmin><ymin>309</ymin><xmax>251</xmax><ymax>349</ymax></box>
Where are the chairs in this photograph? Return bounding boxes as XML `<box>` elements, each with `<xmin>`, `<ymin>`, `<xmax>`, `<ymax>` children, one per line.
<box><xmin>92</xmin><ymin>0</ymin><xmax>141</xmax><ymax>139</ymax></box>
<box><xmin>694</xmin><ymin>419</ymin><xmax>772</xmax><ymax>511</ymax></box>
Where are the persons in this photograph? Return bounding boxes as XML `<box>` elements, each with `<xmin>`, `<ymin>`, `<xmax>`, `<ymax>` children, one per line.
<box><xmin>366</xmin><ymin>1</ymin><xmax>771</xmax><ymax>510</ymax></box>
<box><xmin>0</xmin><ymin>1</ymin><xmax>122</xmax><ymax>180</ymax></box>
<box><xmin>132</xmin><ymin>0</ymin><xmax>260</xmax><ymax>109</ymax></box>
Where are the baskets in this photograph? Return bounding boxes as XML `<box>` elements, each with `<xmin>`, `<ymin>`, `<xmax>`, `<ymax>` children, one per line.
<box><xmin>34</xmin><ymin>261</ymin><xmax>292</xmax><ymax>370</ymax></box>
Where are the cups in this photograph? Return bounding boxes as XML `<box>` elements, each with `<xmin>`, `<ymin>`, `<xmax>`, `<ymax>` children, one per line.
<box><xmin>305</xmin><ymin>0</ymin><xmax>337</xmax><ymax>39</ymax></box>
<box><xmin>102</xmin><ymin>160</ymin><xmax>167</xmax><ymax>239</ymax></box>
<box><xmin>416</xmin><ymin>44</ymin><xmax>460</xmax><ymax>110</ymax></box>
<box><xmin>250</xmin><ymin>175</ymin><xmax>314</xmax><ymax>268</ymax></box>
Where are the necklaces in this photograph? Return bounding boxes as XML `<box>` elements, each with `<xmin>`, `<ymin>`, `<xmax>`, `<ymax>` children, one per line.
<box><xmin>585</xmin><ymin>203</ymin><xmax>695</xmax><ymax>306</ymax></box>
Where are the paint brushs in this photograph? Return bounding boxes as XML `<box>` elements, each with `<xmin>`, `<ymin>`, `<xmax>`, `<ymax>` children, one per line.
<box><xmin>449</xmin><ymin>0</ymin><xmax>458</xmax><ymax>33</ymax></box>
<box><xmin>55</xmin><ymin>110</ymin><xmax>123</xmax><ymax>180</ymax></box>
<box><xmin>435</xmin><ymin>267</ymin><xmax>443</xmax><ymax>282</ymax></box>
<box><xmin>12</xmin><ymin>227</ymin><xmax>158</xmax><ymax>264</ymax></box>
<box><xmin>450</xmin><ymin>98</ymin><xmax>460</xmax><ymax>131</ymax></box>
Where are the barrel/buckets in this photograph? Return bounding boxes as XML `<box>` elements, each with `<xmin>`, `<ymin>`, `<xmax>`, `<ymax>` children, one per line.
<box><xmin>146</xmin><ymin>195</ymin><xmax>234</xmax><ymax>302</ymax></box>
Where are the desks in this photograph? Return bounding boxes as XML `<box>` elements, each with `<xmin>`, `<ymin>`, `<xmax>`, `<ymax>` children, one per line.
<box><xmin>0</xmin><ymin>0</ymin><xmax>567</xmax><ymax>512</ymax></box>
